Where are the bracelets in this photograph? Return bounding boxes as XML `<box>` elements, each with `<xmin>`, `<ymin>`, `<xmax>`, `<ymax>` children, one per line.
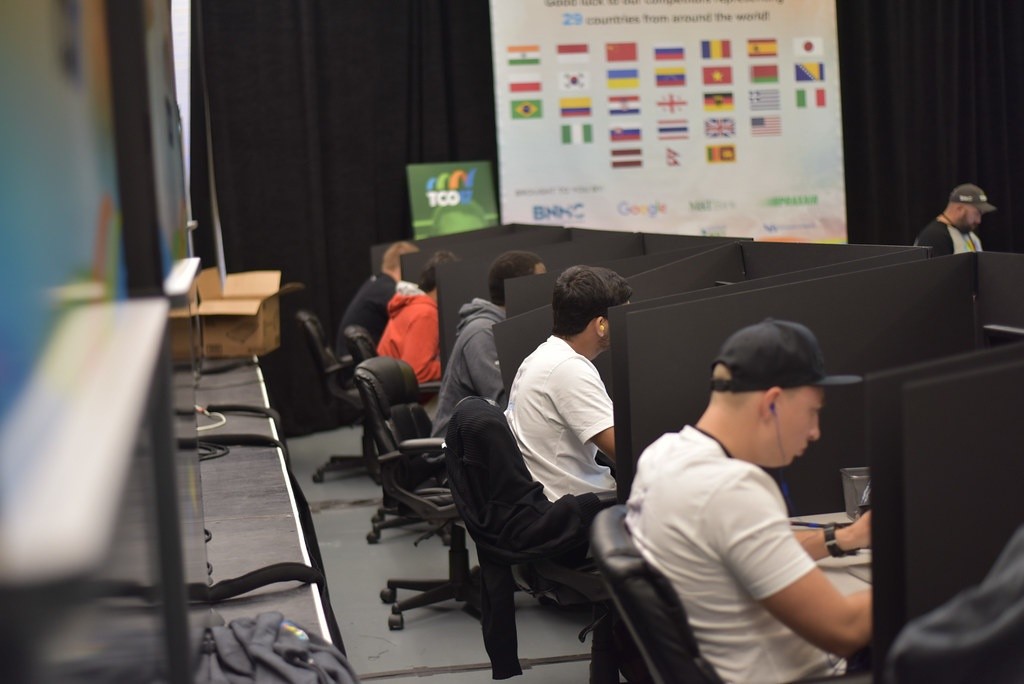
<box><xmin>824</xmin><ymin>521</ymin><xmax>849</xmax><ymax>558</ymax></box>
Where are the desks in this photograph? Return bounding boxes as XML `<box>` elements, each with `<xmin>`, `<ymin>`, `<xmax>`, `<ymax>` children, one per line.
<box><xmin>790</xmin><ymin>512</ymin><xmax>872</xmax><ymax>593</ymax></box>
<box><xmin>194</xmin><ymin>357</ymin><xmax>334</xmax><ymax>646</ymax></box>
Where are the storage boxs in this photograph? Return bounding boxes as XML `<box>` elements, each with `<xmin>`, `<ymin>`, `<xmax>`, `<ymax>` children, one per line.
<box><xmin>195</xmin><ymin>266</ymin><xmax>305</xmax><ymax>359</ymax></box>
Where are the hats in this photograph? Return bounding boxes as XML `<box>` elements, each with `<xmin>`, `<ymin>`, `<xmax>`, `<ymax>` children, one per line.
<box><xmin>707</xmin><ymin>319</ymin><xmax>864</xmax><ymax>392</ymax></box>
<box><xmin>950</xmin><ymin>184</ymin><xmax>997</xmax><ymax>211</ymax></box>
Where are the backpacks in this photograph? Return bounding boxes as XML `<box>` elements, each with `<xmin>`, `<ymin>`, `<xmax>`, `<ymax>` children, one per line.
<box><xmin>195</xmin><ymin>611</ymin><xmax>361</xmax><ymax>684</ymax></box>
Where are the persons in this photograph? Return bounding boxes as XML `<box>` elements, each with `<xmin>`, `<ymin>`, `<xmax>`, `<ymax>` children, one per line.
<box><xmin>336</xmin><ymin>240</ymin><xmax>421</xmax><ymax>364</ymax></box>
<box><xmin>503</xmin><ymin>265</ymin><xmax>632</xmax><ymax>509</ymax></box>
<box><xmin>376</xmin><ymin>250</ymin><xmax>457</xmax><ymax>384</ymax></box>
<box><xmin>625</xmin><ymin>320</ymin><xmax>872</xmax><ymax>683</ymax></box>
<box><xmin>430</xmin><ymin>251</ymin><xmax>547</xmax><ymax>437</ymax></box>
<box><xmin>914</xmin><ymin>184</ymin><xmax>997</xmax><ymax>258</ymax></box>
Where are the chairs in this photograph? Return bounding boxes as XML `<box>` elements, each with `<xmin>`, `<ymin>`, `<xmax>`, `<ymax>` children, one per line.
<box><xmin>588</xmin><ymin>504</ymin><xmax>872</xmax><ymax>684</ymax></box>
<box><xmin>293</xmin><ymin>310</ymin><xmax>383</xmax><ymax>486</ymax></box>
<box><xmin>352</xmin><ymin>355</ymin><xmax>559</xmax><ymax>630</ymax></box>
<box><xmin>442</xmin><ymin>395</ymin><xmax>609</xmax><ymax>684</ymax></box>
<box><xmin>344</xmin><ymin>323</ymin><xmax>442</xmax><ymax>544</ymax></box>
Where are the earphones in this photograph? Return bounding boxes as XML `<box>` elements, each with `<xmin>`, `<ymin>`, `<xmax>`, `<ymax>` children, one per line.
<box><xmin>770</xmin><ymin>403</ymin><xmax>775</xmax><ymax>413</ymax></box>
<box><xmin>599</xmin><ymin>324</ymin><xmax>605</xmax><ymax>332</ymax></box>
